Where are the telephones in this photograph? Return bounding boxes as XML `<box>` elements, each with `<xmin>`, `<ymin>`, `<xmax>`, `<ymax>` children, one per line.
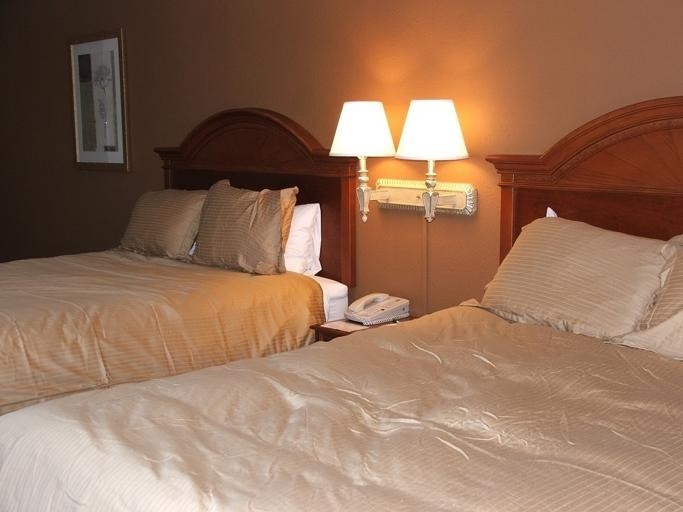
<box><xmin>344</xmin><ymin>292</ymin><xmax>409</xmax><ymax>325</ymax></box>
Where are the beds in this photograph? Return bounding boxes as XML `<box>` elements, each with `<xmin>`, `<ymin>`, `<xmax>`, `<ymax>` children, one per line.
<box><xmin>0</xmin><ymin>95</ymin><xmax>683</xmax><ymax>511</ymax></box>
<box><xmin>0</xmin><ymin>109</ymin><xmax>358</xmax><ymax>414</ymax></box>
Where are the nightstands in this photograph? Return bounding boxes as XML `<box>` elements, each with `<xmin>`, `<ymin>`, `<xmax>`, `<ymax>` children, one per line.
<box><xmin>310</xmin><ymin>317</ymin><xmax>417</xmax><ymax>343</ymax></box>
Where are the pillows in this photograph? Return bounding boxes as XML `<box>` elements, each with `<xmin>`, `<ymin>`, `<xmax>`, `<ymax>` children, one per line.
<box><xmin>604</xmin><ymin>234</ymin><xmax>682</xmax><ymax>358</ymax></box>
<box><xmin>282</xmin><ymin>201</ymin><xmax>322</xmax><ymax>276</ymax></box>
<box><xmin>193</xmin><ymin>180</ymin><xmax>298</xmax><ymax>276</ymax></box>
<box><xmin>118</xmin><ymin>190</ymin><xmax>205</xmax><ymax>264</ymax></box>
<box><xmin>481</xmin><ymin>216</ymin><xmax>674</xmax><ymax>338</ymax></box>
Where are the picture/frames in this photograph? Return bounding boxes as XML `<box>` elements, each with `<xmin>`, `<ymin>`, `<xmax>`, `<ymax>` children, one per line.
<box><xmin>68</xmin><ymin>26</ymin><xmax>132</xmax><ymax>174</ymax></box>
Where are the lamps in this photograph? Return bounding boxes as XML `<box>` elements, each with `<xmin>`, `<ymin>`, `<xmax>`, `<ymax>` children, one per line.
<box><xmin>395</xmin><ymin>99</ymin><xmax>475</xmax><ymax>223</ymax></box>
<box><xmin>328</xmin><ymin>99</ymin><xmax>395</xmax><ymax>224</ymax></box>
